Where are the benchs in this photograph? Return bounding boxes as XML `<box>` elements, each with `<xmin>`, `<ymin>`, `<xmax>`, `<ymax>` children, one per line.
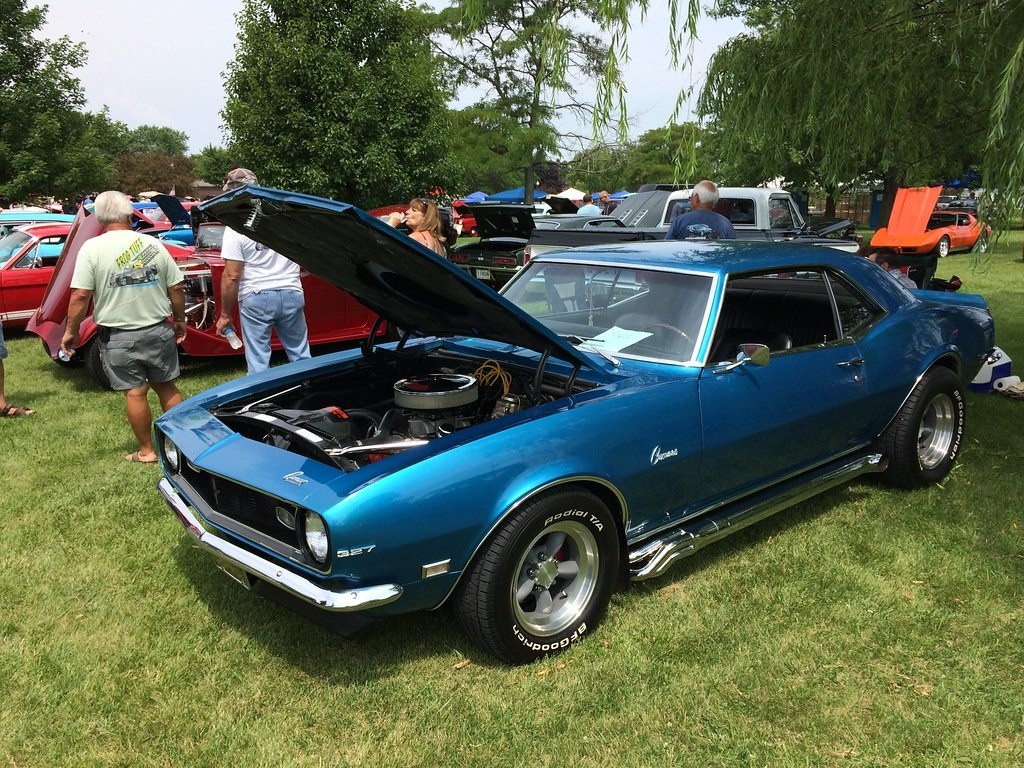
<box><xmin>714</xmin><ymin>289</ymin><xmax>866</xmax><ymax>348</ymax></box>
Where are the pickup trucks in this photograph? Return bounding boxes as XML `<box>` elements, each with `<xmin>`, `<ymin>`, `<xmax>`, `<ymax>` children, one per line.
<box><xmin>524</xmin><ymin>186</ymin><xmax>863</xmax><ymax>294</ymax></box>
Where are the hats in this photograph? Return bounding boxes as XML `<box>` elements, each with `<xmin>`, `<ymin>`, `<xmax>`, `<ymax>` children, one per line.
<box><xmin>222</xmin><ymin>168</ymin><xmax>260</xmax><ymax>192</ymax></box>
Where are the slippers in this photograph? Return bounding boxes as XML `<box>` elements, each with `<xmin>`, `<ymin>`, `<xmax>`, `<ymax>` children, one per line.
<box><xmin>0</xmin><ymin>403</ymin><xmax>36</xmax><ymax>417</ymax></box>
<box><xmin>126</xmin><ymin>449</ymin><xmax>158</xmax><ymax>463</ymax></box>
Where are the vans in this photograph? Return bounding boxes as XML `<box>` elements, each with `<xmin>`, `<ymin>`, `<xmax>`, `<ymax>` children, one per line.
<box><xmin>937</xmin><ymin>195</ymin><xmax>957</xmax><ymax>209</ymax></box>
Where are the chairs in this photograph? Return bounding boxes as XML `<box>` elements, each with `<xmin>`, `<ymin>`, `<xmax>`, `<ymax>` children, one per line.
<box><xmin>707</xmin><ymin>326</ymin><xmax>793</xmax><ymax>363</ymax></box>
<box><xmin>542</xmin><ymin>264</ymin><xmax>603</xmax><ymax>314</ymax></box>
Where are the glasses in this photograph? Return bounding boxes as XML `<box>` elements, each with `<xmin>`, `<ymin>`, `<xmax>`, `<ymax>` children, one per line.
<box><xmin>420</xmin><ymin>198</ymin><xmax>428</xmax><ymax>209</ymax></box>
<box><xmin>689</xmin><ymin>194</ymin><xmax>696</xmax><ymax>199</ymax></box>
<box><xmin>600</xmin><ymin>196</ymin><xmax>605</xmax><ymax>200</ymax></box>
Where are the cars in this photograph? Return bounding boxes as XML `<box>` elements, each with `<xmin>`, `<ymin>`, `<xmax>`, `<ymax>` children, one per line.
<box><xmin>597</xmin><ymin>198</ymin><xmax>624</xmax><ymax>214</ymax></box>
<box><xmin>451</xmin><ymin>183</ymin><xmax>671</xmax><ymax>290</ymax></box>
<box><xmin>153</xmin><ymin>183</ymin><xmax>997</xmax><ymax>665</ymax></box>
<box><xmin>532</xmin><ymin>203</ymin><xmax>553</xmax><ymax>216</ymax></box>
<box><xmin>24</xmin><ymin>202</ymin><xmax>419</xmax><ymax>389</ymax></box>
<box><xmin>946</xmin><ymin>198</ymin><xmax>987</xmax><ymax>218</ymax></box>
<box><xmin>0</xmin><ymin>199</ymin><xmax>208</xmax><ymax>330</ymax></box>
<box><xmin>452</xmin><ymin>201</ymin><xmax>478</xmax><ymax>239</ymax></box>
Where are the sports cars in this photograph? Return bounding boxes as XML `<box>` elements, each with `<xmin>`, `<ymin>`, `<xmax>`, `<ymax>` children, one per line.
<box><xmin>869</xmin><ymin>183</ymin><xmax>992</xmax><ymax>257</ymax></box>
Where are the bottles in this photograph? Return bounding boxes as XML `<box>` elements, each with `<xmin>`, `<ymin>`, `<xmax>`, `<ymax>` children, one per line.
<box><xmin>223</xmin><ymin>326</ymin><xmax>243</xmax><ymax>349</ymax></box>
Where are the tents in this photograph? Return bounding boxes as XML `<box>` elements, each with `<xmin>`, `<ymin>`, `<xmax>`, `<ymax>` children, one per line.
<box><xmin>757</xmin><ymin>167</ymin><xmax>982</xmax><ymax>220</ymax></box>
<box><xmin>462</xmin><ymin>184</ymin><xmax>634</xmax><ymax>205</ymax></box>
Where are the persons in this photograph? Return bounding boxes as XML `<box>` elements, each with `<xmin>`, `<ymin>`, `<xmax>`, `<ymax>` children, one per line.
<box><xmin>388</xmin><ymin>198</ymin><xmax>447</xmax><ymax>260</ymax></box>
<box><xmin>576</xmin><ymin>190</ymin><xmax>618</xmax><ymax>215</ymax></box>
<box><xmin>866</xmin><ymin>247</ymin><xmax>916</xmax><ymax>288</ymax></box>
<box><xmin>660</xmin><ymin>179</ymin><xmax>737</xmax><ymax>240</ymax></box>
<box><xmin>0</xmin><ymin>167</ymin><xmax>305</xmax><ymax>464</ymax></box>
